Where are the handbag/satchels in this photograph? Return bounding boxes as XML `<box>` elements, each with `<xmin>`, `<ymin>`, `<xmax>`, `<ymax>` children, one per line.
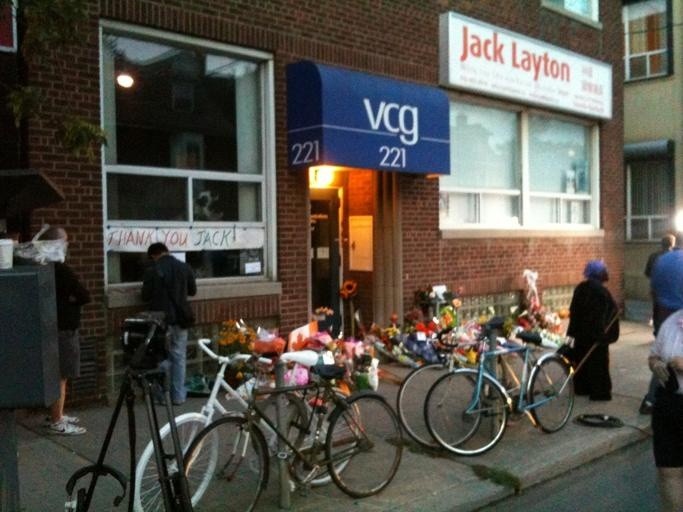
<box><xmin>173</xmin><ymin>297</ymin><xmax>199</xmax><ymax>330</ymax></box>
<box><xmin>556</xmin><ymin>344</ymin><xmax>572</xmax><ymax>358</ymax></box>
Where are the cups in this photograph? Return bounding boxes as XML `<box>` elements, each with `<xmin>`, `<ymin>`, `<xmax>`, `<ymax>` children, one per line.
<box><xmin>0</xmin><ymin>239</ymin><xmax>14</xmax><ymax>269</ymax></box>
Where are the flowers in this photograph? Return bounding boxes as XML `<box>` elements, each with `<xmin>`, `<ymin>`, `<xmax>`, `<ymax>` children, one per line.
<box><xmin>211</xmin><ymin>317</ymin><xmax>286</xmax><ymax>388</ymax></box>
<box><xmin>309</xmin><ymin>278</ymin><xmax>358</xmax><ymax>331</ymax></box>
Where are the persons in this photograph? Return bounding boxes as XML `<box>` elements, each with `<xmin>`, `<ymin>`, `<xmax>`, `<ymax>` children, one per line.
<box><xmin>647</xmin><ymin>306</ymin><xmax>683</xmax><ymax>512</ymax></box>
<box><xmin>139</xmin><ymin>242</ymin><xmax>197</xmax><ymax>405</ymax></box>
<box><xmin>566</xmin><ymin>258</ymin><xmax>620</xmax><ymax>402</ymax></box>
<box><xmin>35</xmin><ymin>227</ymin><xmax>91</xmax><ymax>437</ymax></box>
<box><xmin>643</xmin><ymin>233</ymin><xmax>676</xmax><ymax>339</ymax></box>
<box><xmin>638</xmin><ymin>229</ymin><xmax>682</xmax><ymax>415</ymax></box>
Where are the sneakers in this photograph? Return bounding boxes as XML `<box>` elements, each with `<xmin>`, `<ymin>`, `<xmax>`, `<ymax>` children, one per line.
<box><xmin>638</xmin><ymin>397</ymin><xmax>652</xmax><ymax>415</ymax></box>
<box><xmin>45</xmin><ymin>414</ymin><xmax>86</xmax><ymax>436</ymax></box>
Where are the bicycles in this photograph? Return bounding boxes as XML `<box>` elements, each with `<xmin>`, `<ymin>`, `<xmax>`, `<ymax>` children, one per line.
<box><xmin>398</xmin><ymin>317</ymin><xmax>575</xmax><ymax>456</ymax></box>
